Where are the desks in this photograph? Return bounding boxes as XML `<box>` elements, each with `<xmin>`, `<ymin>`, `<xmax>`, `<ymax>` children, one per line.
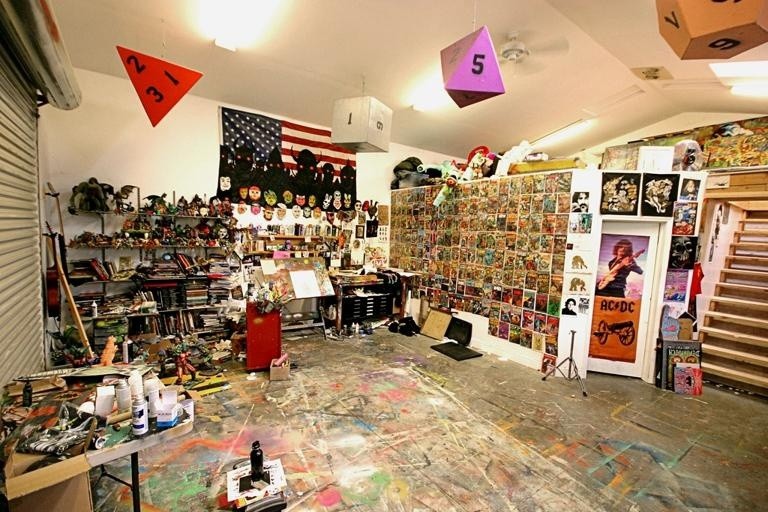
<box><xmin>322</xmin><ymin>271</ymin><xmax>408</xmax><ymax>331</ymax></box>
<box><xmin>0</xmin><ymin>364</ymin><xmax>195</xmax><ymax>512</ymax></box>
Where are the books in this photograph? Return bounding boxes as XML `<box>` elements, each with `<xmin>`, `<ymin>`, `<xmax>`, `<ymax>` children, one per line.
<box><xmin>227</xmin><ymin>458</ymin><xmax>288</xmax><ymax>512</ymax></box>
<box><xmin>63</xmin><ymin>253</ymin><xmax>235</xmax><ymax>375</ymax></box>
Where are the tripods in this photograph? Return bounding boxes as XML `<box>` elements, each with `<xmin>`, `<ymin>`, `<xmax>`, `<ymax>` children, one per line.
<box><xmin>542</xmin><ymin>330</ymin><xmax>588</xmax><ymax>397</ymax></box>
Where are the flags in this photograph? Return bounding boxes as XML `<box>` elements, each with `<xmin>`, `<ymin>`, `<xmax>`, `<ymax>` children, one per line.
<box><xmin>216</xmin><ymin>106</ymin><xmax>356</xmax><ymax>212</ymax></box>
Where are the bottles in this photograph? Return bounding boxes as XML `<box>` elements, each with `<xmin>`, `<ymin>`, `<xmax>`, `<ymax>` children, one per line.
<box><xmin>22</xmin><ymin>376</ymin><xmax>33</xmax><ymax>406</ymax></box>
<box><xmin>90</xmin><ymin>300</ymin><xmax>98</xmax><ymax>318</ymax></box>
<box><xmin>250</xmin><ymin>439</ymin><xmax>263</xmax><ymax>482</ymax></box>
<box><xmin>115</xmin><ymin>369</ymin><xmax>160</xmax><ymax>437</ymax></box>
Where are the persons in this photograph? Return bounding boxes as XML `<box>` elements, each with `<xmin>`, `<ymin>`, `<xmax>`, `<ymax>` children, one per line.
<box><xmin>562</xmin><ymin>298</ymin><xmax>577</xmax><ymax>315</ymax></box>
<box><xmin>595</xmin><ymin>238</ymin><xmax>643</xmax><ymax>297</ymax></box>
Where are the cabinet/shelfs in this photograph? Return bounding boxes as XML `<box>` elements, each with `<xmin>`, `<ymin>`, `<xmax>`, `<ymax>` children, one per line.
<box><xmin>65</xmin><ymin>212</ymin><xmax>229</xmax><ymax>354</ymax></box>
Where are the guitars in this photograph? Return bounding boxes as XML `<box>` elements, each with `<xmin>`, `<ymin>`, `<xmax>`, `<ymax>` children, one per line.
<box><xmin>47</xmin><ymin>182</ymin><xmax>71</xmax><ymax>284</ymax></box>
<box><xmin>598</xmin><ymin>248</ymin><xmax>644</xmax><ymax>290</ymax></box>
<box><xmin>45</xmin><ymin>220</ymin><xmax>61</xmax><ymax>318</ymax></box>
